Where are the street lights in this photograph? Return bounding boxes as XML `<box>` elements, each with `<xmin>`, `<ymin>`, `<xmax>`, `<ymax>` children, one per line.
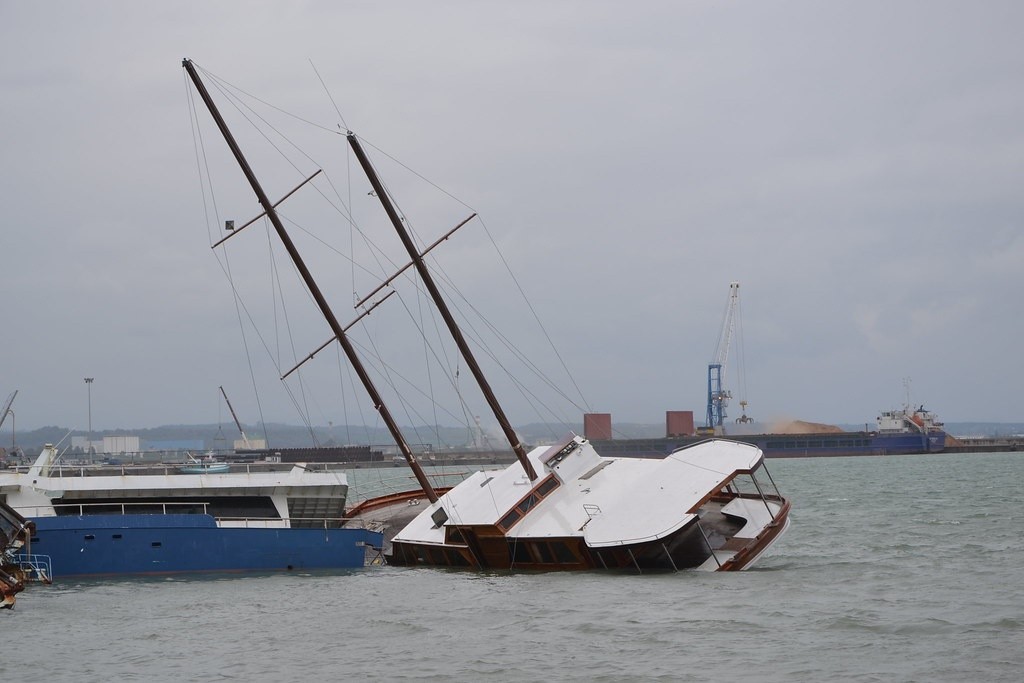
<box><xmin>84</xmin><ymin>375</ymin><xmax>98</xmax><ymax>457</ymax></box>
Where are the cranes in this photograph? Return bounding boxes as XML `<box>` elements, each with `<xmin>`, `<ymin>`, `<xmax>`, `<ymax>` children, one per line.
<box><xmin>707</xmin><ymin>281</ymin><xmax>755</xmax><ymax>427</ymax></box>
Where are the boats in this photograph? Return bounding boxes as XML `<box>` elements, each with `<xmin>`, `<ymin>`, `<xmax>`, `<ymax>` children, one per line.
<box><xmin>181</xmin><ymin>56</ymin><xmax>792</xmax><ymax>577</ymax></box>
<box><xmin>1</xmin><ymin>442</ymin><xmax>385</xmax><ymax>580</ymax></box>
